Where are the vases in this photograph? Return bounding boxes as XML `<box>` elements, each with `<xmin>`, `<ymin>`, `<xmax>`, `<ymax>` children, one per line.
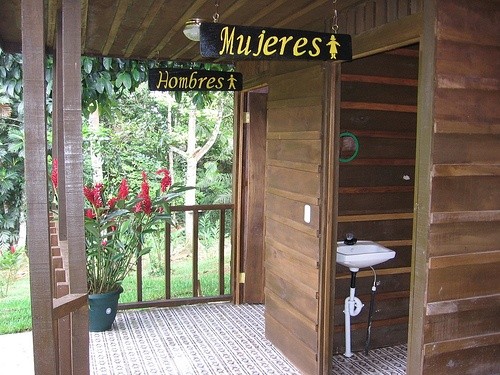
<box><xmin>88</xmin><ymin>283</ymin><xmax>123</xmax><ymax>332</ymax></box>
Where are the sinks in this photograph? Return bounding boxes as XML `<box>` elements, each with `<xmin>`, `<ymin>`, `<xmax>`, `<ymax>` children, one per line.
<box><xmin>330</xmin><ymin>239</ymin><xmax>396</xmax><ymax>270</ymax></box>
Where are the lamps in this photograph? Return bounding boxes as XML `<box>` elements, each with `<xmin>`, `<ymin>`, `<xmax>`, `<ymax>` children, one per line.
<box><xmin>183</xmin><ymin>18</ymin><xmax>211</xmax><ymax>42</ymax></box>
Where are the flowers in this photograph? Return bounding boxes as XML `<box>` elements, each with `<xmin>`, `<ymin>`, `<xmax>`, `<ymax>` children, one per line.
<box><xmin>50</xmin><ymin>159</ymin><xmax>199</xmax><ymax>294</ymax></box>
